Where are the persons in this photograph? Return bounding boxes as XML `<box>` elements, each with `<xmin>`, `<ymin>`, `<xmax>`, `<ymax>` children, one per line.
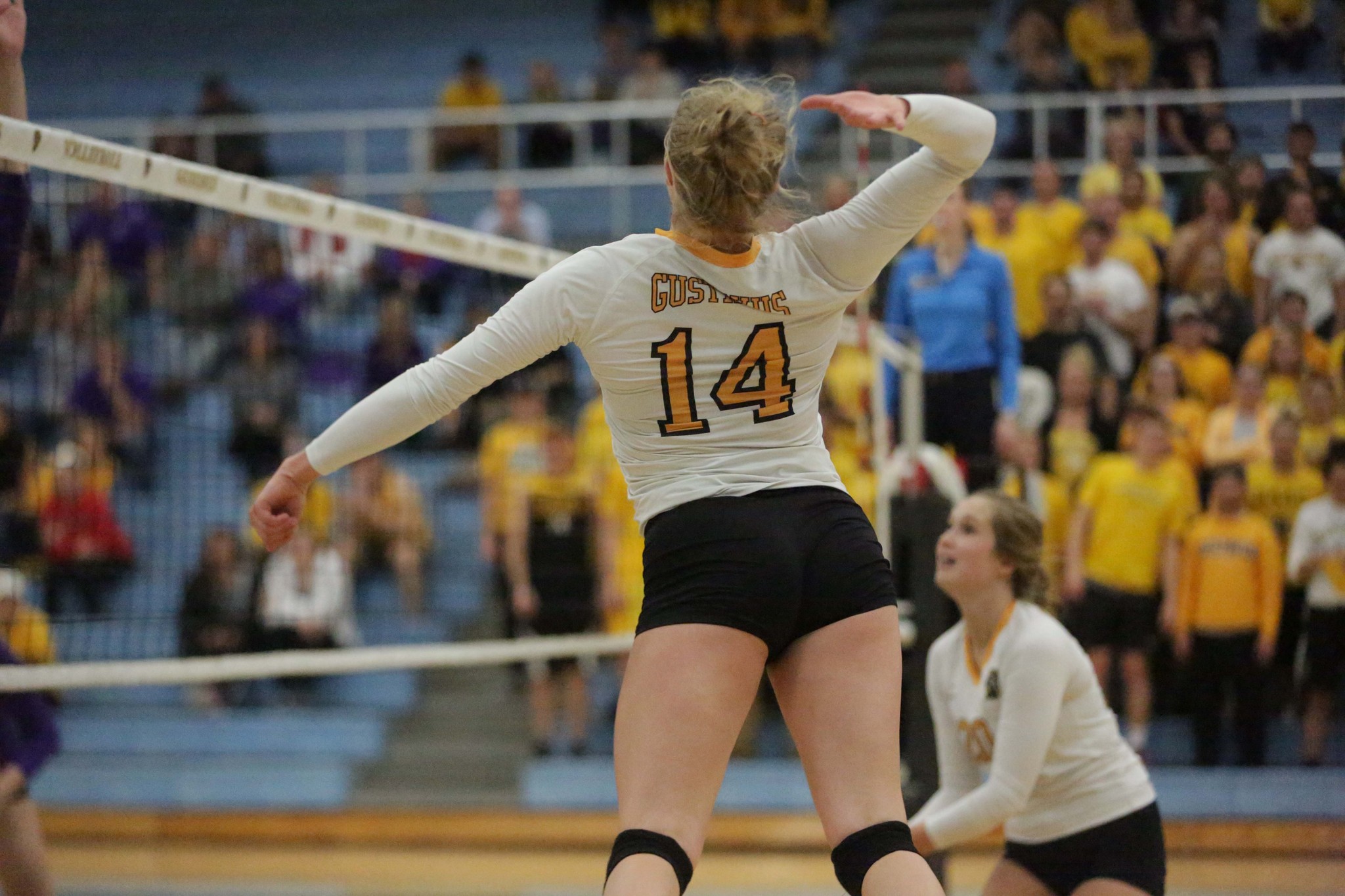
<box><xmin>0</xmin><ymin>0</ymin><xmax>1345</xmax><ymax>896</ymax></box>
<box><xmin>906</xmin><ymin>487</ymin><xmax>1166</xmax><ymax>896</ymax></box>
<box><xmin>250</xmin><ymin>71</ymin><xmax>997</xmax><ymax>896</ymax></box>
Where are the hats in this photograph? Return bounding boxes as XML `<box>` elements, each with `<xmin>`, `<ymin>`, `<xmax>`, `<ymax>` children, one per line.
<box><xmin>1168</xmin><ymin>297</ymin><xmax>1202</xmax><ymax>322</ymax></box>
